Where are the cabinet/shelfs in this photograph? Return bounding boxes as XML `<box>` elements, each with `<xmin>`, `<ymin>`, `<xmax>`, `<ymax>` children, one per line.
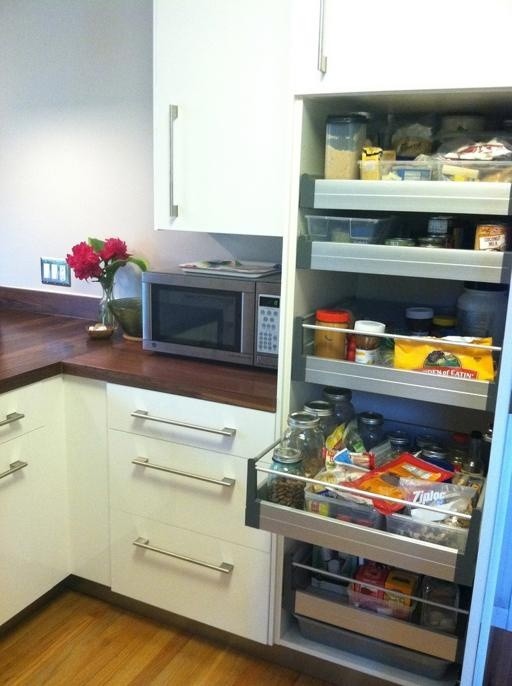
<box><xmin>240</xmin><ymin>82</ymin><xmax>509</xmax><ymax>681</ymax></box>
<box><xmin>99</xmin><ymin>378</ymin><xmax>277</xmax><ymax>665</ymax></box>
<box><xmin>1</xmin><ymin>378</ymin><xmax>67</xmax><ymax>642</ymax></box>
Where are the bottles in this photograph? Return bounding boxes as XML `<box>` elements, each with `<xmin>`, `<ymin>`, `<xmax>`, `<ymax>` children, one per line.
<box><xmin>267</xmin><ymin>388</ymin><xmax>384</xmax><ymax>510</ymax></box>
<box><xmin>448</xmin><ymin>430</ymin><xmax>485</xmax><ymax>490</ymax></box>
<box><xmin>98</xmin><ymin>284</ymin><xmax>119</xmax><ymax>330</ymax></box>
<box><xmin>458</xmin><ymin>279</ymin><xmax>509</xmax><ymax>348</ymax></box>
<box><xmin>323</xmin><ymin>112</ymin><xmax>366</xmax><ymax>180</ymax></box>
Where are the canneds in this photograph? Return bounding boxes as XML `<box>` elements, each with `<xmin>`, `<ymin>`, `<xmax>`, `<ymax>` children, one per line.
<box><xmin>384</xmin><ymin>216</ymin><xmax>511</xmax><ymax>252</ymax></box>
<box><xmin>313</xmin><ymin>308</ymin><xmax>349</xmax><ymax>361</ymax></box>
<box><xmin>353</xmin><ymin>319</ymin><xmax>385</xmax><ymax>366</ymax></box>
<box><xmin>267</xmin><ymin>387</ymin><xmax>447</xmax><ymax>508</ymax></box>
<box><xmin>406</xmin><ymin>307</ymin><xmax>458</xmax><ymax>337</ymax></box>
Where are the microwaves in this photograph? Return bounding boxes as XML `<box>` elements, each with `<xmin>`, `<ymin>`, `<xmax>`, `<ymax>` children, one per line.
<box><xmin>140</xmin><ymin>257</ymin><xmax>280</xmax><ymax>370</ymax></box>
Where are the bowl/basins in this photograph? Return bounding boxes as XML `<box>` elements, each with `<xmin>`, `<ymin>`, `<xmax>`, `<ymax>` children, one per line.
<box><xmin>107</xmin><ymin>297</ymin><xmax>142</xmax><ymax>336</ymax></box>
<box><xmin>86</xmin><ymin>329</ymin><xmax>113</xmax><ymax>339</ymax></box>
